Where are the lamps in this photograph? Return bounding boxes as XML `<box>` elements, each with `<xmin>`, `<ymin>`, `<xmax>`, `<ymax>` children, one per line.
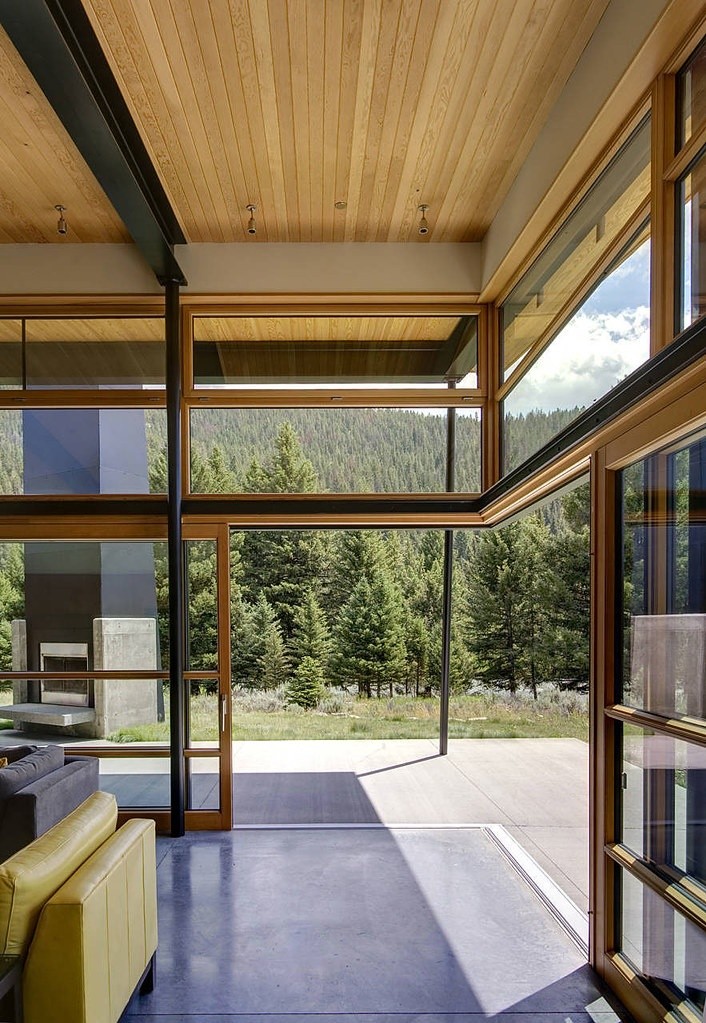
<box><xmin>55</xmin><ymin>205</ymin><xmax>67</xmax><ymax>233</ymax></box>
<box><xmin>246</xmin><ymin>204</ymin><xmax>256</xmax><ymax>234</ymax></box>
<box><xmin>417</xmin><ymin>204</ymin><xmax>430</xmax><ymax>234</ymax></box>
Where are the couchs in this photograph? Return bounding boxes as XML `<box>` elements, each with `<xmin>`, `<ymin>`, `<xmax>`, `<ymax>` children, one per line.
<box><xmin>0</xmin><ymin>744</ymin><xmax>158</xmax><ymax>1023</ymax></box>
